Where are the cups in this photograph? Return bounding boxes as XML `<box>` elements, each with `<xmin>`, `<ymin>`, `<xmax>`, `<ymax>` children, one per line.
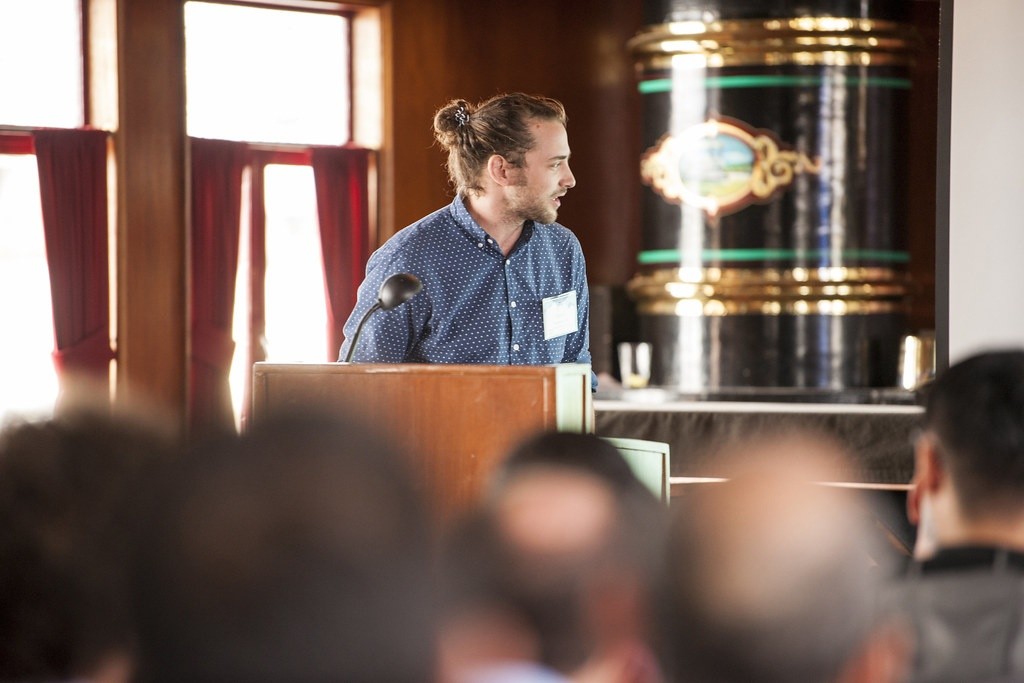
<box><xmin>618</xmin><ymin>342</ymin><xmax>654</xmax><ymax>389</ymax></box>
<box><xmin>899</xmin><ymin>331</ymin><xmax>937</xmax><ymax>391</ymax></box>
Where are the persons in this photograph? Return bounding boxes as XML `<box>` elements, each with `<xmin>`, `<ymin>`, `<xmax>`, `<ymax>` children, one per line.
<box><xmin>0</xmin><ymin>410</ymin><xmax>173</xmax><ymax>683</ymax></box>
<box><xmin>639</xmin><ymin>422</ymin><xmax>912</xmax><ymax>683</ymax></box>
<box><xmin>429</xmin><ymin>430</ymin><xmax>659</xmax><ymax>683</ymax></box>
<box><xmin>900</xmin><ymin>347</ymin><xmax>1024</xmax><ymax>682</ymax></box>
<box><xmin>118</xmin><ymin>400</ymin><xmax>439</xmax><ymax>683</ymax></box>
<box><xmin>337</xmin><ymin>92</ymin><xmax>598</xmax><ymax>392</ymax></box>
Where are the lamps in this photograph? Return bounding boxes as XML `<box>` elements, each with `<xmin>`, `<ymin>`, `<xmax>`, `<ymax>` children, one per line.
<box><xmin>343</xmin><ymin>273</ymin><xmax>426</xmax><ymax>363</ymax></box>
